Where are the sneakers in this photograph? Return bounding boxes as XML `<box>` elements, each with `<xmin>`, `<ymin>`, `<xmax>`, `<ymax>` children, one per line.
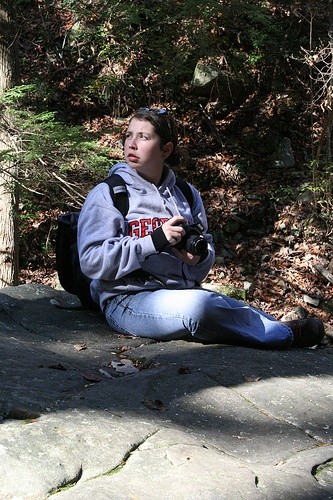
<box><xmin>279</xmin><ymin>306</ymin><xmax>305</xmax><ymax>322</ymax></box>
<box><xmin>282</xmin><ymin>318</ymin><xmax>325</xmax><ymax>349</ymax></box>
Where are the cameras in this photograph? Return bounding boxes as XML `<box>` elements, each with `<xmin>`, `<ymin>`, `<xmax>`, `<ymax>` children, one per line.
<box><xmin>176</xmin><ymin>222</ymin><xmax>208</xmax><ymax>255</ymax></box>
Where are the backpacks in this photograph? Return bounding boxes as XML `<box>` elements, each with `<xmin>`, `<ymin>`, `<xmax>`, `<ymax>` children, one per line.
<box><xmin>56</xmin><ymin>172</ymin><xmax>194</xmax><ymax>307</ymax></box>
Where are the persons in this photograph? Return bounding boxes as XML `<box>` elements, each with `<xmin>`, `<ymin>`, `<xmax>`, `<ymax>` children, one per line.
<box><xmin>77</xmin><ymin>110</ymin><xmax>325</xmax><ymax>351</ymax></box>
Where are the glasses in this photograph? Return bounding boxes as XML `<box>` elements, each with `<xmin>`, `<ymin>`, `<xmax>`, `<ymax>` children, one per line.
<box><xmin>136</xmin><ymin>107</ymin><xmax>172</xmax><ymax>142</ymax></box>
